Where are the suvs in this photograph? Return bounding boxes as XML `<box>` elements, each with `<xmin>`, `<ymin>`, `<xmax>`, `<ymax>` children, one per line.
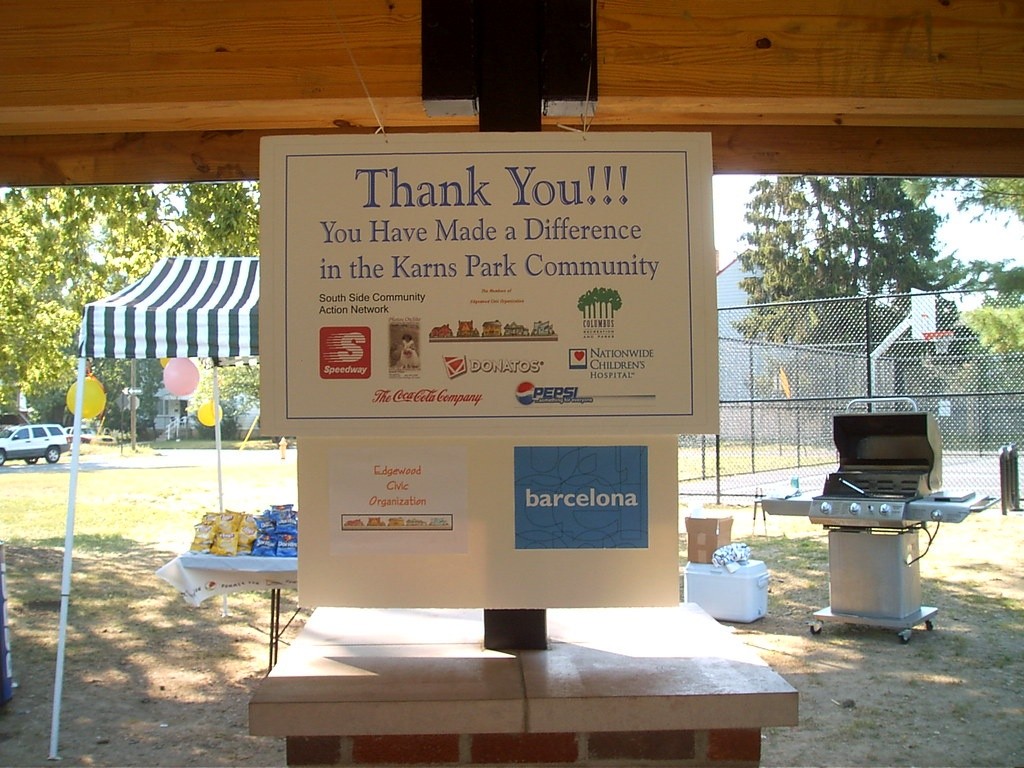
<box><xmin>0</xmin><ymin>424</ymin><xmax>71</xmax><ymax>469</ymax></box>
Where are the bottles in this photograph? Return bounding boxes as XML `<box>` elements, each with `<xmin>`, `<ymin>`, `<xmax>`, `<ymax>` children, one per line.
<box><xmin>791</xmin><ymin>476</ymin><xmax>798</xmax><ymax>488</ymax></box>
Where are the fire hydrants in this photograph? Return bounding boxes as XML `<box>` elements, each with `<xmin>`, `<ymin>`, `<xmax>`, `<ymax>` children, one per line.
<box><xmin>278</xmin><ymin>436</ymin><xmax>287</xmax><ymax>462</ymax></box>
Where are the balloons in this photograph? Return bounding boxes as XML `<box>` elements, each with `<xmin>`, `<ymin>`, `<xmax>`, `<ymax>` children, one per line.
<box><xmin>65</xmin><ymin>377</ymin><xmax>107</xmax><ymax>418</ymax></box>
<box><xmin>161</xmin><ymin>358</ymin><xmax>200</xmax><ymax>396</ymax></box>
<box><xmin>198</xmin><ymin>399</ymin><xmax>223</xmax><ymax>427</ymax></box>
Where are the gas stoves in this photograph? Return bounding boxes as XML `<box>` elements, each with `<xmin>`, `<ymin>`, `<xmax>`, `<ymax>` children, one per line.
<box><xmin>808</xmin><ymin>488</ymin><xmax>932</xmax><ymax>523</ymax></box>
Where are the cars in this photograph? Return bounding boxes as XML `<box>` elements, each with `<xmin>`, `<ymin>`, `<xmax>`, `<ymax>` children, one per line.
<box><xmin>63</xmin><ymin>425</ymin><xmax>116</xmax><ymax>446</ymax></box>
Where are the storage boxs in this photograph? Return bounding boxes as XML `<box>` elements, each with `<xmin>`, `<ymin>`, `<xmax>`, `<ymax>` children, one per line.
<box><xmin>685</xmin><ymin>517</ymin><xmax>735</xmax><ymax>564</ymax></box>
<box><xmin>684</xmin><ymin>559</ymin><xmax>770</xmax><ymax>624</ymax></box>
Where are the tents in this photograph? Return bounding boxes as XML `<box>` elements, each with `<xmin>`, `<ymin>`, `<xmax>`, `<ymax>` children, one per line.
<box><xmin>47</xmin><ymin>253</ymin><xmax>259</xmax><ymax>762</ymax></box>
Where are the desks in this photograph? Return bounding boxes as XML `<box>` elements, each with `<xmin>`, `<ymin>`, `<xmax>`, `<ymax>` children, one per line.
<box><xmin>156</xmin><ymin>553</ymin><xmax>301</xmax><ymax>674</ymax></box>
